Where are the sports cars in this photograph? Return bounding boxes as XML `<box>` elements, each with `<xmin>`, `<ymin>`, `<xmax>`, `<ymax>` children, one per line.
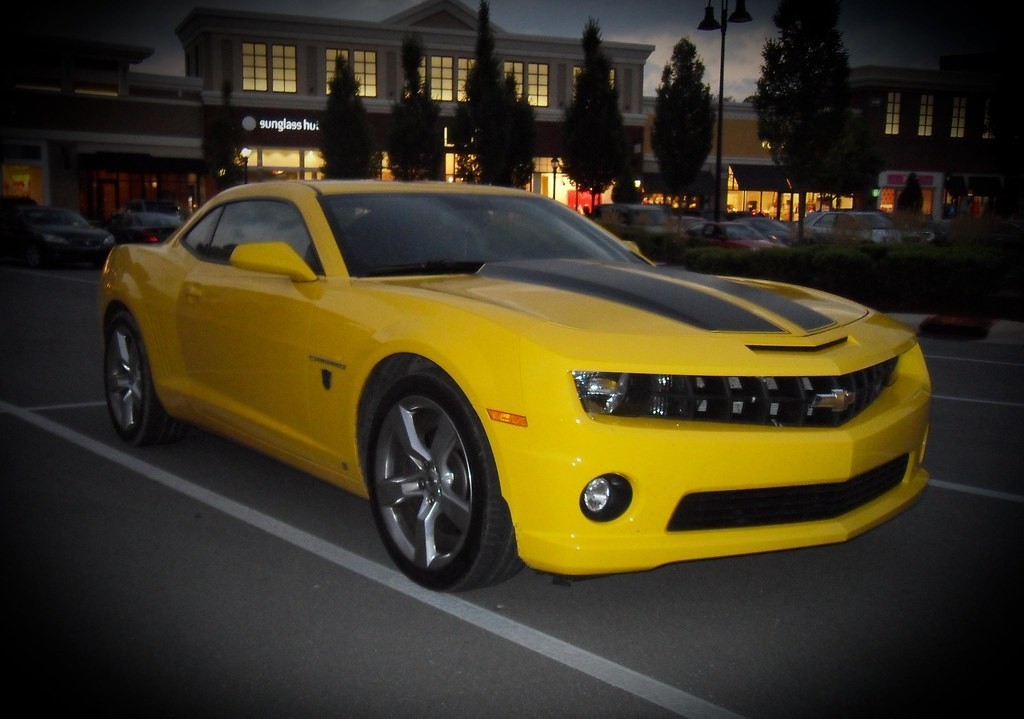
<box><xmin>98</xmin><ymin>179</ymin><xmax>932</xmax><ymax>592</ymax></box>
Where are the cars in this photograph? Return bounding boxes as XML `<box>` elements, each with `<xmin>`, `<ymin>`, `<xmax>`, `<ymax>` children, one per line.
<box><xmin>110</xmin><ymin>198</ymin><xmax>186</xmax><ymax>242</ymax></box>
<box><xmin>2</xmin><ymin>205</ymin><xmax>116</xmax><ymax>272</ymax></box>
<box><xmin>587</xmin><ymin>205</ymin><xmax>935</xmax><ymax>262</ymax></box>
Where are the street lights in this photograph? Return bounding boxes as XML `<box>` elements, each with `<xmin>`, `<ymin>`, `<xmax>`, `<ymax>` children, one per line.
<box><xmin>550</xmin><ymin>152</ymin><xmax>559</xmax><ymax>201</ymax></box>
<box><xmin>698</xmin><ymin>0</ymin><xmax>752</xmax><ymax>230</ymax></box>
<box><xmin>240</xmin><ymin>145</ymin><xmax>251</xmax><ymax>184</ymax></box>
